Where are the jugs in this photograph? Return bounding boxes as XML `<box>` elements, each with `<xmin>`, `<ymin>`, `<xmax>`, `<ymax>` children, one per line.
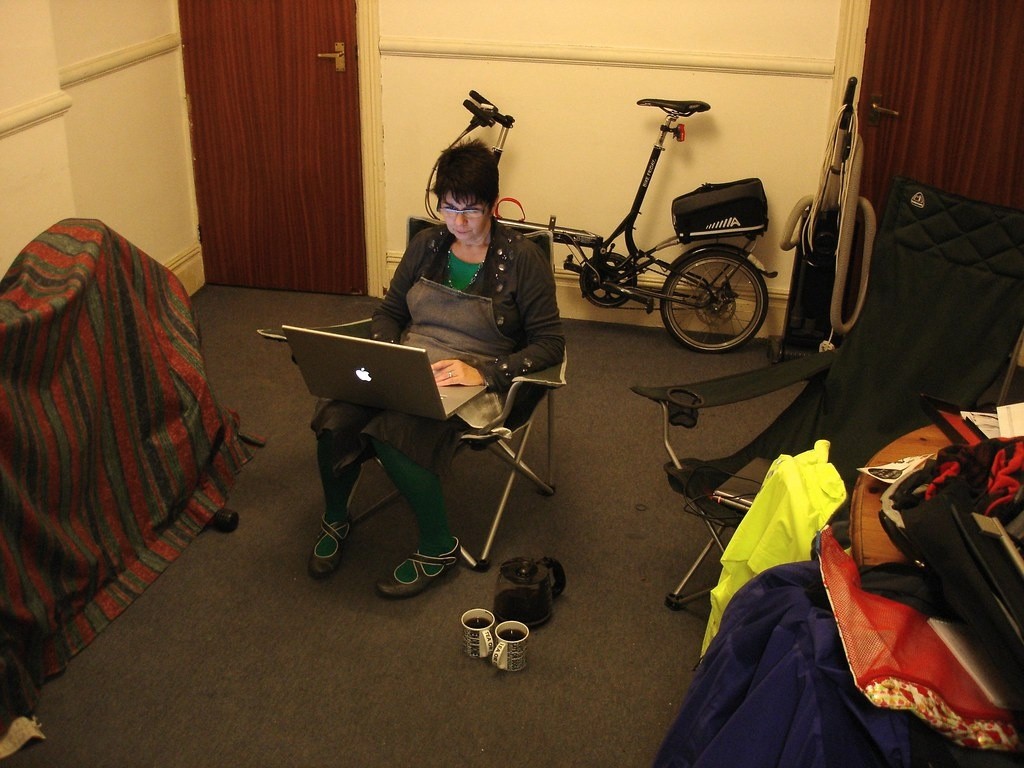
<box><xmin>493</xmin><ymin>556</ymin><xmax>566</xmax><ymax>628</ymax></box>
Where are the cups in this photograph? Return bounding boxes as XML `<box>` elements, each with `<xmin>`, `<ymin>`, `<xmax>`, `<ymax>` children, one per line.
<box><xmin>492</xmin><ymin>621</ymin><xmax>530</xmax><ymax>671</ymax></box>
<box><xmin>461</xmin><ymin>608</ymin><xmax>495</xmax><ymax>659</ymax></box>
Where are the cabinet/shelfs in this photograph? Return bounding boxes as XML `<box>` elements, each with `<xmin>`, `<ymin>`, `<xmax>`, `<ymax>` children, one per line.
<box><xmin>826</xmin><ymin>0</ymin><xmax>1024</xmax><ymax>322</ymax></box>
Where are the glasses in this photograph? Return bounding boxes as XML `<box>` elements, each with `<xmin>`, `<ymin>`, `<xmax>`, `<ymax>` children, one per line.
<box><xmin>436</xmin><ymin>197</ymin><xmax>490</xmax><ymax>217</ymax></box>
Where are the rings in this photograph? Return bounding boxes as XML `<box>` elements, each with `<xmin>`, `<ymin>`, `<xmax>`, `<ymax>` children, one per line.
<box><xmin>449</xmin><ymin>372</ymin><xmax>452</xmax><ymax>377</ymax></box>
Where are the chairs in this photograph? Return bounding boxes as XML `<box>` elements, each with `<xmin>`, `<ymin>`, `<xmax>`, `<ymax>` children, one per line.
<box><xmin>256</xmin><ymin>215</ymin><xmax>568</xmax><ymax>573</ymax></box>
<box><xmin>627</xmin><ymin>174</ymin><xmax>1024</xmax><ymax>611</ymax></box>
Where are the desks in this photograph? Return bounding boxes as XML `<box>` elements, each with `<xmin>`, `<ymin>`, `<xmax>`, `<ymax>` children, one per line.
<box><xmin>849</xmin><ymin>423</ymin><xmax>950</xmax><ymax>565</ymax></box>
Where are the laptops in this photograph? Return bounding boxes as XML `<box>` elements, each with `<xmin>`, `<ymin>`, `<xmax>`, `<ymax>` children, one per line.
<box><xmin>713</xmin><ymin>457</ymin><xmax>773</xmax><ymax>512</ymax></box>
<box><xmin>282</xmin><ymin>325</ymin><xmax>486</xmax><ymax>421</ymax></box>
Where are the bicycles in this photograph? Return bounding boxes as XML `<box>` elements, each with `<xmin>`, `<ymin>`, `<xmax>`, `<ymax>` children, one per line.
<box><xmin>421</xmin><ymin>89</ymin><xmax>781</xmax><ymax>355</ymax></box>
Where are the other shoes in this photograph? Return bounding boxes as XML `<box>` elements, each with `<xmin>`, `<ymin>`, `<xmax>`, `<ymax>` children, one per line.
<box><xmin>378</xmin><ymin>536</ymin><xmax>461</xmax><ymax>596</ymax></box>
<box><xmin>310</xmin><ymin>509</ymin><xmax>352</xmax><ymax>577</ymax></box>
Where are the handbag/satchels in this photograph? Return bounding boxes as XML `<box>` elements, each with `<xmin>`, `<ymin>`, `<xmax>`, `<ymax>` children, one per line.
<box><xmin>882</xmin><ymin>432</ymin><xmax>1023</xmax><ymax>704</ymax></box>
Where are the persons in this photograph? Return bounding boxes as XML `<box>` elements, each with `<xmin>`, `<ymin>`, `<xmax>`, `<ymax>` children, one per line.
<box><xmin>307</xmin><ymin>139</ymin><xmax>566</xmax><ymax>598</ymax></box>
